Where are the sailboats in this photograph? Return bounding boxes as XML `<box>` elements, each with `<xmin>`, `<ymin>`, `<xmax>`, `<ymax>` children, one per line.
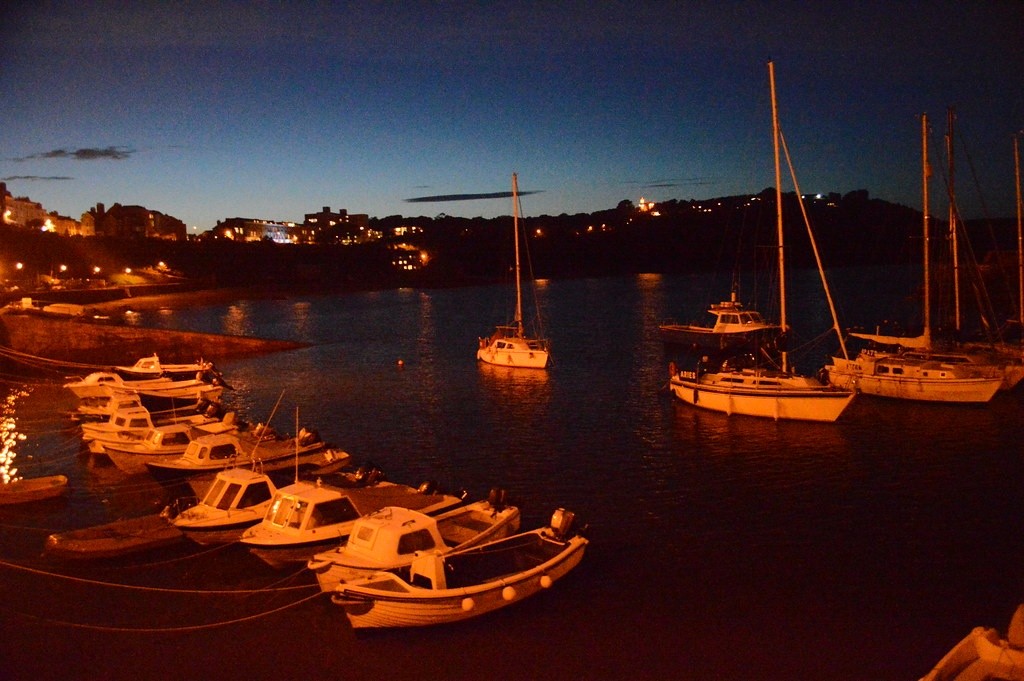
<box><xmin>849</xmin><ymin>113</ymin><xmax>1024</xmax><ymax>391</ymax></box>
<box><xmin>773</xmin><ymin>121</ymin><xmax>1010</xmax><ymax>406</ymax></box>
<box><xmin>666</xmin><ymin>58</ymin><xmax>861</xmax><ymax>424</ymax></box>
<box><xmin>473</xmin><ymin>171</ymin><xmax>554</xmax><ymax>369</ymax></box>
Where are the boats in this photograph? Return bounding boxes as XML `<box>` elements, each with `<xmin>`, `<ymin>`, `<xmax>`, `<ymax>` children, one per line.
<box><xmin>922</xmin><ymin>602</ymin><xmax>1024</xmax><ymax>681</ymax></box>
<box><xmin>73</xmin><ymin>361</ymin><xmax>383</xmax><ymax>530</ymax></box>
<box><xmin>329</xmin><ymin>502</ymin><xmax>594</xmax><ymax>629</ymax></box>
<box><xmin>110</xmin><ymin>354</ymin><xmax>228</xmax><ymax>384</ymax></box>
<box><xmin>237</xmin><ymin>402</ymin><xmax>470</xmax><ymax>571</ymax></box>
<box><xmin>311</xmin><ymin>486</ymin><xmax>522</xmax><ymax>595</ymax></box>
<box><xmin>653</xmin><ymin>271</ymin><xmax>781</xmax><ymax>352</ymax></box>
<box><xmin>62</xmin><ymin>369</ymin><xmax>240</xmax><ymax>404</ymax></box>
<box><xmin>0</xmin><ymin>473</ymin><xmax>70</xmax><ymax>506</ymax></box>
<box><xmin>47</xmin><ymin>504</ymin><xmax>185</xmax><ymax>563</ymax></box>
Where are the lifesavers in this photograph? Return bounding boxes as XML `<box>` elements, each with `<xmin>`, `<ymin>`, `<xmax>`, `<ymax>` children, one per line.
<box><xmin>669</xmin><ymin>362</ymin><xmax>677</xmax><ymax>376</ymax></box>
<box><xmin>774</xmin><ymin>332</ymin><xmax>793</xmax><ymax>352</ymax></box>
